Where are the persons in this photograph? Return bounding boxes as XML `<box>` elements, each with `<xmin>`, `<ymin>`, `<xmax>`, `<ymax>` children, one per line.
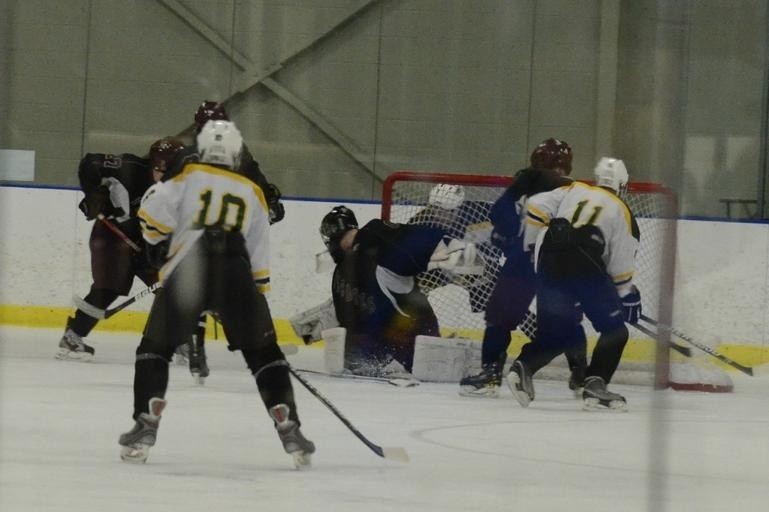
<box><xmin>459</xmin><ymin>138</ymin><xmax>590</xmax><ymax>389</ymax></box>
<box><xmin>119</xmin><ymin>120</ymin><xmax>315</xmax><ymax>453</ymax></box>
<box><xmin>406</xmin><ymin>183</ymin><xmax>539</xmax><ymax>347</ymax></box>
<box><xmin>59</xmin><ymin>137</ymin><xmax>197</xmax><ymax>360</ymax></box>
<box><xmin>319</xmin><ymin>205</ymin><xmax>486</xmax><ymax>384</ymax></box>
<box><xmin>161</xmin><ymin>100</ymin><xmax>285</xmax><ymax>376</ymax></box>
<box><xmin>510</xmin><ymin>157</ymin><xmax>642</xmax><ymax>408</ymax></box>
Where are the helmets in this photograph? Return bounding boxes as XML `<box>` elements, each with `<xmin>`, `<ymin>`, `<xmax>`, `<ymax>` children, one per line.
<box><xmin>193</xmin><ymin>116</ymin><xmax>245</xmax><ymax>172</ymax></box>
<box><xmin>591</xmin><ymin>155</ymin><xmax>630</xmax><ymax>197</ymax></box>
<box><xmin>148</xmin><ymin>137</ymin><xmax>187</xmax><ymax>173</ymax></box>
<box><xmin>429</xmin><ymin>180</ymin><xmax>465</xmax><ymax>211</ymax></box>
<box><xmin>193</xmin><ymin>100</ymin><xmax>229</xmax><ymax>123</ymax></box>
<box><xmin>318</xmin><ymin>204</ymin><xmax>359</xmax><ymax>264</ymax></box>
<box><xmin>530</xmin><ymin>137</ymin><xmax>573</xmax><ymax>175</ymax></box>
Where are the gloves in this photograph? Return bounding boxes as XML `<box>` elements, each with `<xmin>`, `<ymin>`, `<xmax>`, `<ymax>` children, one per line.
<box><xmin>78</xmin><ymin>186</ymin><xmax>114</xmax><ymax>222</ymax></box>
<box><xmin>262</xmin><ymin>184</ymin><xmax>285</xmax><ymax>225</ymax></box>
<box><xmin>617</xmin><ymin>284</ymin><xmax>645</xmax><ymax>326</ymax></box>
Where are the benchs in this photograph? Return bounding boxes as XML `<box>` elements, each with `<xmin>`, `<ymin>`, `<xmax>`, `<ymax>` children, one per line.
<box><xmin>719</xmin><ymin>197</ymin><xmax>766</xmax><ymax>219</ymax></box>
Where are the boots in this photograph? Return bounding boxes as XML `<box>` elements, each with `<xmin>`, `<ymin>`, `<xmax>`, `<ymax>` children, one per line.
<box><xmin>269</xmin><ymin>404</ymin><xmax>316</xmax><ymax>455</ymax></box>
<box><xmin>118</xmin><ymin>398</ymin><xmax>167</xmax><ymax>447</ymax></box>
<box><xmin>58</xmin><ymin>316</ymin><xmax>95</xmax><ymax>356</ymax></box>
<box><xmin>187</xmin><ymin>334</ymin><xmax>213</xmax><ymax>378</ymax></box>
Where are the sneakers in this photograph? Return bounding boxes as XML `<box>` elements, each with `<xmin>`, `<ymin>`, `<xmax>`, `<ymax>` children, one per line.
<box><xmin>509</xmin><ymin>359</ymin><xmax>538</xmax><ymax>402</ymax></box>
<box><xmin>568</xmin><ymin>367</ymin><xmax>627</xmax><ymax>404</ymax></box>
<box><xmin>459</xmin><ymin>362</ymin><xmax>504</xmax><ymax>389</ymax></box>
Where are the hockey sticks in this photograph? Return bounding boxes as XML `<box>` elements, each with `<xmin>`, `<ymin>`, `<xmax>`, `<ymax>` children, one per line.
<box><xmin>71</xmin><ymin>282</ymin><xmax>164</xmax><ymax>321</ymax></box>
<box><xmin>287</xmin><ymin>363</ymin><xmax>408</xmax><ymax>462</ymax></box>
<box><xmin>632</xmin><ymin>315</ymin><xmax>769</xmax><ymax>378</ymax></box>
